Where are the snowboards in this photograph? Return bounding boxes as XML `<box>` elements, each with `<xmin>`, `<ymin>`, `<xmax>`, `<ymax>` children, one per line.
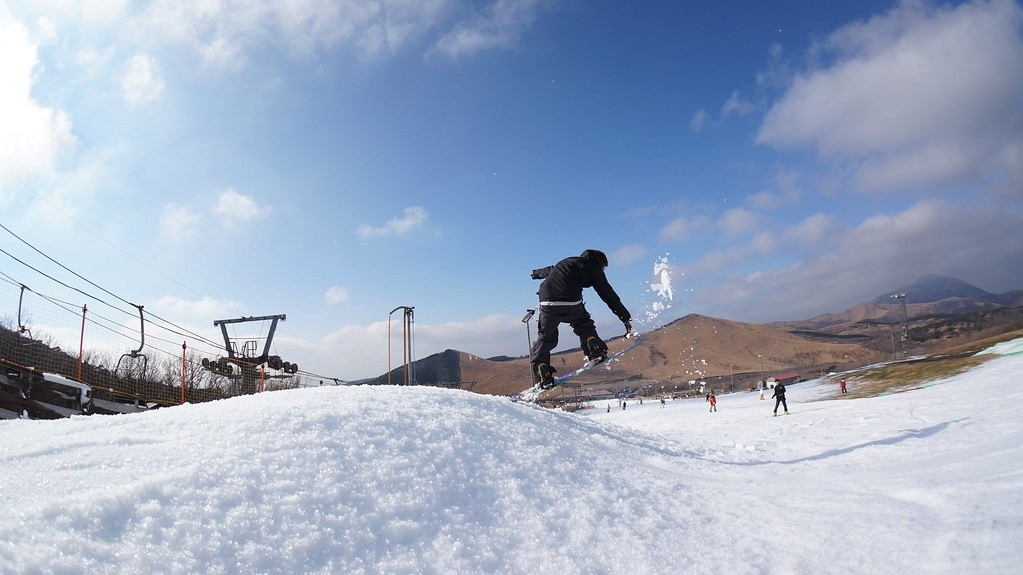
<box><xmin>533</xmin><ymin>337</ymin><xmax>646</xmax><ymax>394</ymax></box>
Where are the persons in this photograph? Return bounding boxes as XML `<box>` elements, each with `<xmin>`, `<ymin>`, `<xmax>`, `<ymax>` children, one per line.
<box><xmin>660</xmin><ymin>397</ymin><xmax>666</xmax><ymax>405</ymax></box>
<box><xmin>706</xmin><ymin>393</ymin><xmax>710</xmax><ymax>402</ymax></box>
<box><xmin>640</xmin><ymin>398</ymin><xmax>643</xmax><ymax>405</ymax></box>
<box><xmin>840</xmin><ymin>378</ymin><xmax>848</xmax><ymax>393</ymax></box>
<box><xmin>607</xmin><ymin>403</ymin><xmax>610</xmax><ymax>412</ymax></box>
<box><xmin>709</xmin><ymin>390</ymin><xmax>717</xmax><ymax>412</ymax></box>
<box><xmin>530</xmin><ymin>249</ymin><xmax>632</xmax><ymax>389</ymax></box>
<box><xmin>623</xmin><ymin>402</ymin><xmax>626</xmax><ymax>410</ymax></box>
<box><xmin>772</xmin><ymin>379</ymin><xmax>788</xmax><ymax>416</ymax></box>
<box><xmin>760</xmin><ymin>387</ymin><xmax>765</xmax><ymax>400</ymax></box>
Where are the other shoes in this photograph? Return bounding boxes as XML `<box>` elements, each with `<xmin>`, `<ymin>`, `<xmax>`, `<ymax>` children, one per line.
<box><xmin>785</xmin><ymin>411</ymin><xmax>788</xmax><ymax>414</ymax></box>
<box><xmin>586</xmin><ymin>336</ymin><xmax>604</xmax><ymax>361</ymax></box>
<box><xmin>774</xmin><ymin>412</ymin><xmax>776</xmax><ymax>415</ymax></box>
<box><xmin>536</xmin><ymin>362</ymin><xmax>555</xmax><ymax>390</ymax></box>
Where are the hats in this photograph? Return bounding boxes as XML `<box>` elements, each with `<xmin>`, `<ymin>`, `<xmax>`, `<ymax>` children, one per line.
<box><xmin>593</xmin><ymin>250</ymin><xmax>608</xmax><ymax>266</ymax></box>
